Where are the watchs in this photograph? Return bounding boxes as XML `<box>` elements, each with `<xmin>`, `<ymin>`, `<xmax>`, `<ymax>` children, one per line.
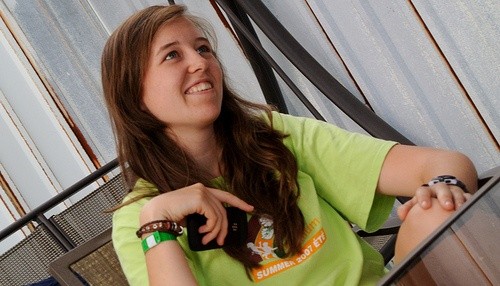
<box><xmin>420</xmin><ymin>175</ymin><xmax>469</xmax><ymax>192</ymax></box>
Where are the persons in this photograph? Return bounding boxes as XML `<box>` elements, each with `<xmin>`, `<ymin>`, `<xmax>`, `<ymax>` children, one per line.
<box><xmin>102</xmin><ymin>5</ymin><xmax>477</xmax><ymax>286</ymax></box>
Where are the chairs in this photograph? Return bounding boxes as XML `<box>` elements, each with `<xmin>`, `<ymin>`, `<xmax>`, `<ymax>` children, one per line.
<box><xmin>45</xmin><ymin>225</ymin><xmax>131</xmax><ymax>286</ymax></box>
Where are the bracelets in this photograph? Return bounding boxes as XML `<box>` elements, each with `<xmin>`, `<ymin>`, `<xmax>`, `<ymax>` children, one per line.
<box><xmin>137</xmin><ymin>220</ymin><xmax>184</xmax><ymax>237</ymax></box>
<box><xmin>141</xmin><ymin>231</ymin><xmax>176</xmax><ymax>252</ymax></box>
